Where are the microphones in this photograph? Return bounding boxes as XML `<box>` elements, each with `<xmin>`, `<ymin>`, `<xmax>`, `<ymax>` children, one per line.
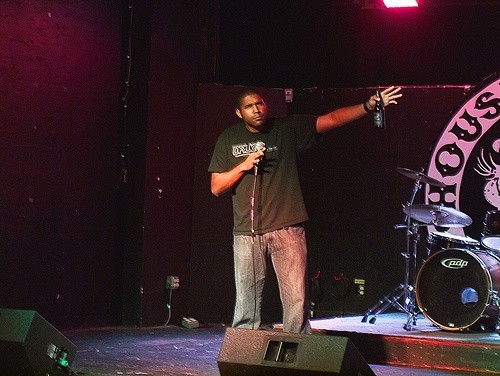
<box><xmin>254</xmin><ymin>141</ymin><xmax>266</xmax><ymax>169</ymax></box>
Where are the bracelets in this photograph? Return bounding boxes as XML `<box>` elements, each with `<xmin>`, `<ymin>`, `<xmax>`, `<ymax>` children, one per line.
<box><xmin>363</xmin><ymin>100</ymin><xmax>373</xmax><ymax>113</ymax></box>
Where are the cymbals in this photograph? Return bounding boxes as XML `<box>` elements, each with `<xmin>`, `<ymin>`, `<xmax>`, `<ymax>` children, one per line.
<box><xmin>397</xmin><ymin>167</ymin><xmax>447</xmax><ymax>187</ymax></box>
<box><xmin>404</xmin><ymin>204</ymin><xmax>473</xmax><ymax>227</ymax></box>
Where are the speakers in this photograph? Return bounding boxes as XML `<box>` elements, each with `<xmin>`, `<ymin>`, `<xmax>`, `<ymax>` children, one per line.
<box><xmin>0</xmin><ymin>308</ymin><xmax>78</xmax><ymax>376</ymax></box>
<box><xmin>217</xmin><ymin>327</ymin><xmax>376</xmax><ymax>376</ymax></box>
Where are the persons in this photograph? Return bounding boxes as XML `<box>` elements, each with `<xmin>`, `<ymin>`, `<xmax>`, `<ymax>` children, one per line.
<box><xmin>207</xmin><ymin>86</ymin><xmax>402</xmax><ymax>336</ymax></box>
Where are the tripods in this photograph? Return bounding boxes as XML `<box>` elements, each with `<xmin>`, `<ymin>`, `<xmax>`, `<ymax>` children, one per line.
<box><xmin>361</xmin><ymin>184</ymin><xmax>422</xmax><ymax>331</ymax></box>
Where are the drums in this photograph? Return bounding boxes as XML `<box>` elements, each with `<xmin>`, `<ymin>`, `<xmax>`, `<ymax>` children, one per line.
<box><xmin>427</xmin><ymin>231</ymin><xmax>479</xmax><ymax>256</ymax></box>
<box><xmin>481</xmin><ymin>235</ymin><xmax>500</xmax><ymax>258</ymax></box>
<box><xmin>415</xmin><ymin>248</ymin><xmax>500</xmax><ymax>332</ymax></box>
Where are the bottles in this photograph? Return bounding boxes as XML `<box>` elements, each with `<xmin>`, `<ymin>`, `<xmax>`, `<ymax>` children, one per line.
<box><xmin>373</xmin><ymin>90</ymin><xmax>385</xmax><ymax>131</ymax></box>
